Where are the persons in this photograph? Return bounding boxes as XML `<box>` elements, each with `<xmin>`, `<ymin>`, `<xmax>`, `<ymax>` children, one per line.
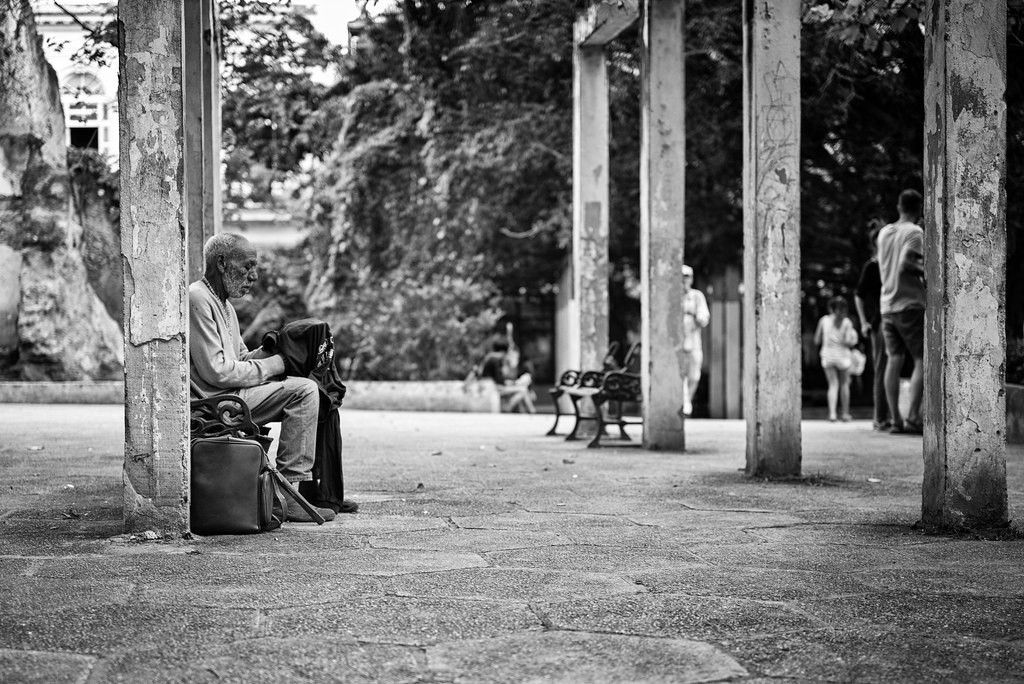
<box><xmin>854</xmin><ymin>228</ymin><xmax>893</xmax><ymax>431</ymax></box>
<box><xmin>877</xmin><ymin>191</ymin><xmax>924</xmax><ymax>433</ymax></box>
<box><xmin>681</xmin><ymin>264</ymin><xmax>711</xmax><ymax>415</ymax></box>
<box><xmin>189</xmin><ymin>232</ymin><xmax>336</xmax><ymax>522</ymax></box>
<box><xmin>480</xmin><ymin>339</ymin><xmax>536</xmax><ymax>415</ymax></box>
<box><xmin>815</xmin><ymin>299</ymin><xmax>858</xmax><ymax>421</ymax></box>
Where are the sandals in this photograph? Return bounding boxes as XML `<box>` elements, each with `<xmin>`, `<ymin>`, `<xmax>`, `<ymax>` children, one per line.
<box><xmin>890</xmin><ymin>418</ymin><xmax>923</xmax><ymax>434</ymax></box>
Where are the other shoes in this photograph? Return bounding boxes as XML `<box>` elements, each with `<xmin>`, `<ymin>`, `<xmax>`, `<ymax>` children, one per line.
<box><xmin>273</xmin><ymin>497</ymin><xmax>336</xmax><ymax>522</ymax></box>
<box><xmin>316</xmin><ymin>498</ymin><xmax>358</xmax><ymax>512</ymax></box>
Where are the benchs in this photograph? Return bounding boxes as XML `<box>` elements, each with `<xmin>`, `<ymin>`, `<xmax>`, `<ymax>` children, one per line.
<box><xmin>546</xmin><ymin>341</ymin><xmax>643</xmax><ymax>448</ymax></box>
<box><xmin>190</xmin><ymin>379</ymin><xmax>326</xmax><ymax>527</ymax></box>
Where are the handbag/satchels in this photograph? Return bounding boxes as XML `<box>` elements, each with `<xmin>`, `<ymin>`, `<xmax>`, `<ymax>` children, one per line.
<box><xmin>191</xmin><ymin>438</ymin><xmax>288</xmax><ymax>534</ymax></box>
<box><xmin>850</xmin><ymin>348</ymin><xmax>867</xmax><ymax>376</ymax></box>
